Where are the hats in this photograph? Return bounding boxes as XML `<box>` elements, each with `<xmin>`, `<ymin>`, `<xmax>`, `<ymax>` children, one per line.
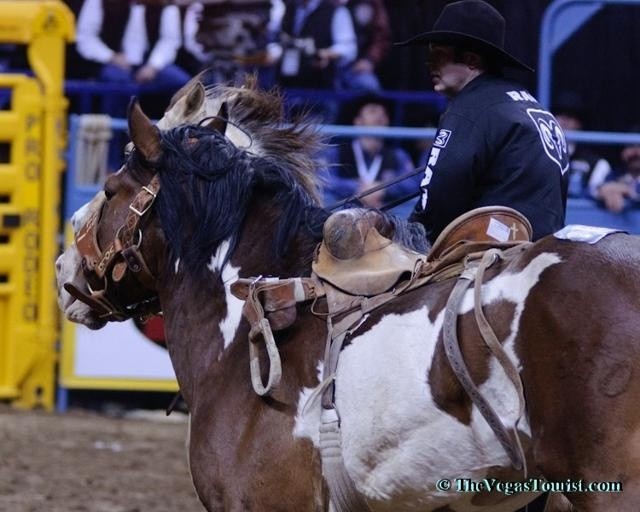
<box><xmin>391</xmin><ymin>2</ymin><xmax>535</xmax><ymax>82</ymax></box>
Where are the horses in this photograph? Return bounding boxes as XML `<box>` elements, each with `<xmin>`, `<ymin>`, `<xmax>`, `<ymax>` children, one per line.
<box><xmin>53</xmin><ymin>65</ymin><xmax>431</xmax><ymax>330</ymax></box>
<box><xmin>72</xmin><ymin>81</ymin><xmax>639</xmax><ymax>512</ymax></box>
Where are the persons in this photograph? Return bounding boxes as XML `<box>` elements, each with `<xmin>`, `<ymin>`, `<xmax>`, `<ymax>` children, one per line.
<box><xmin>405</xmin><ymin>0</ymin><xmax>572</xmax><ymax>246</ymax></box>
<box><xmin>72</xmin><ymin>1</ymin><xmax>640</xmax><ymax>215</ymax></box>
